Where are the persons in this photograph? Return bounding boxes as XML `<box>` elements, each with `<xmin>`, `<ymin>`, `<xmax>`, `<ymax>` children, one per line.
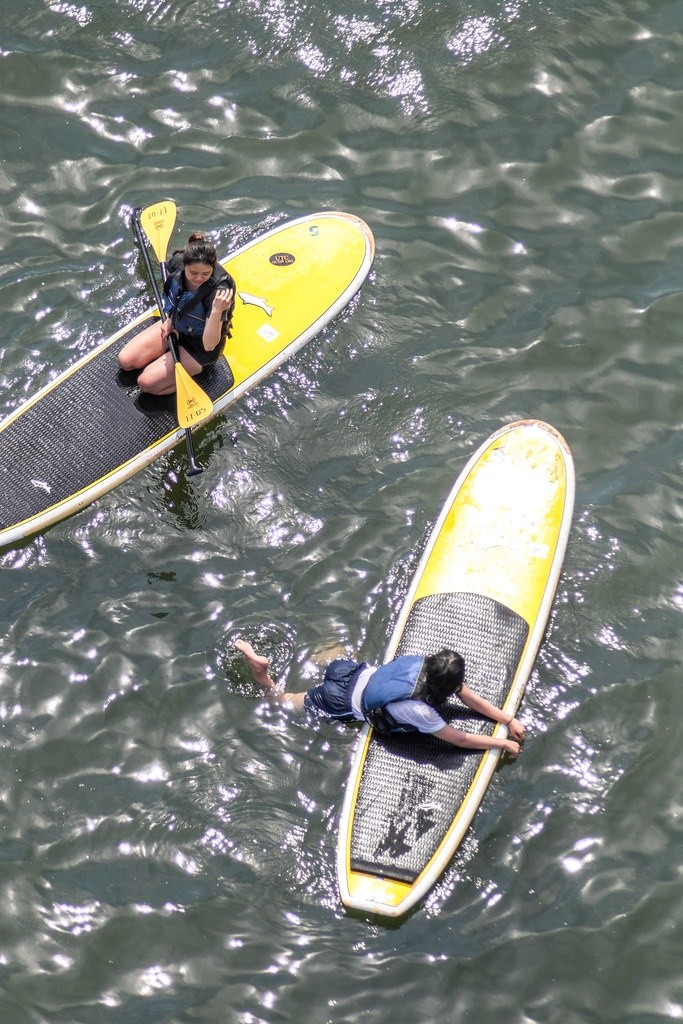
<box><xmin>118</xmin><ymin>234</ymin><xmax>236</xmax><ymax>395</ymax></box>
<box><xmin>234</xmin><ymin>638</ymin><xmax>525</xmax><ymax>760</ymax></box>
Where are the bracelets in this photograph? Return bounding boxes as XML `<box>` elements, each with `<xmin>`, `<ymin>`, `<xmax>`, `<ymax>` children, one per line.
<box><xmin>506</xmin><ymin>718</ymin><xmax>513</xmax><ymax>725</ymax></box>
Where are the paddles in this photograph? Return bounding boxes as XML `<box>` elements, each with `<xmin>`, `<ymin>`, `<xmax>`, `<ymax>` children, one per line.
<box><xmin>131</xmin><ymin>209</ymin><xmax>216</xmax><ymax>431</ymax></box>
<box><xmin>129</xmin><ymin>201</ymin><xmax>205</xmax><ymax>477</ymax></box>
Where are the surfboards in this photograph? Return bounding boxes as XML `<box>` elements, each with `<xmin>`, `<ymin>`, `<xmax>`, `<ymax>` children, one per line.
<box><xmin>336</xmin><ymin>418</ymin><xmax>578</xmax><ymax>917</ymax></box>
<box><xmin>0</xmin><ymin>208</ymin><xmax>379</xmax><ymax>546</ymax></box>
